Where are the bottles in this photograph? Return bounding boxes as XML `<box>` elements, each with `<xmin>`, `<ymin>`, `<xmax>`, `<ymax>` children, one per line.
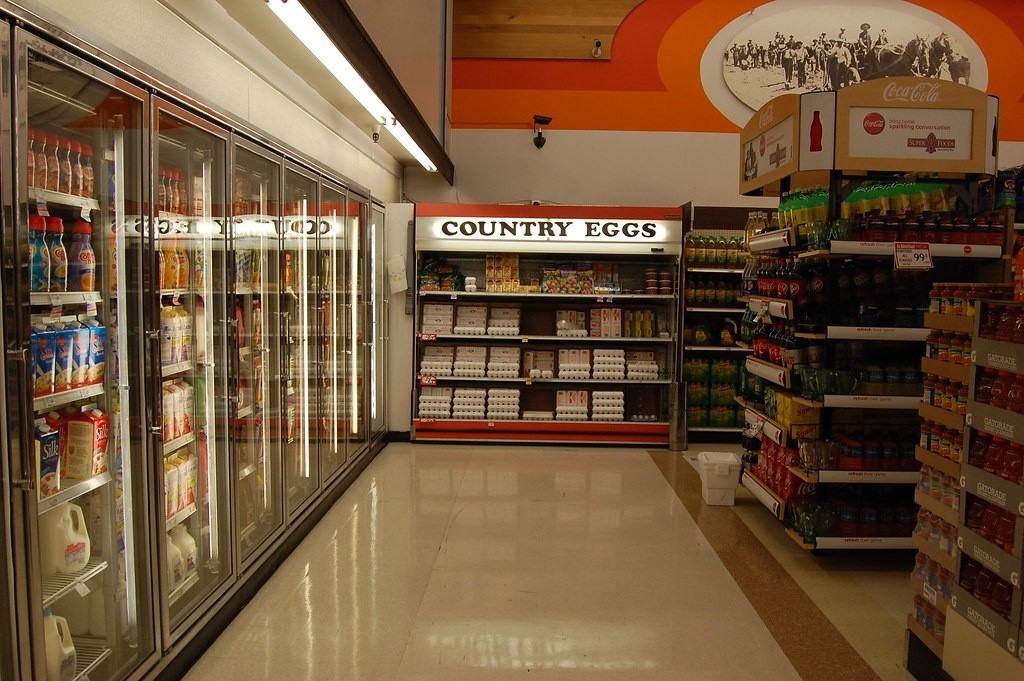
<box><xmin>26</xmin><ymin>125</ymin><xmax>198</xmax><ymax>681</ymax></box>
<box><xmin>683</xmin><ymin>171</ymin><xmax>1024</xmax><ymax>647</ymax></box>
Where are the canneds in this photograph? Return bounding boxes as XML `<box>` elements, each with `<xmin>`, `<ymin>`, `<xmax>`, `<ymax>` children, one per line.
<box><xmin>912</xmin><ymin>280</ymin><xmax>1013</xmax><ymax>645</ymax></box>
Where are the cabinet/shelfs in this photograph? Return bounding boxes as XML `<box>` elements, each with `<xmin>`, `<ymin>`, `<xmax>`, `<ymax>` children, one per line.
<box><xmin>417</xmin><ymin>289</ymin><xmax>675</xmax><ymax>423</ymax></box>
<box><xmin>681</xmin><ymin>177</ymin><xmax>1024</xmax><ymax>681</ymax></box>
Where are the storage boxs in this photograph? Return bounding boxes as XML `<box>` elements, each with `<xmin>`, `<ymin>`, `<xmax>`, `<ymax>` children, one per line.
<box><xmin>696</xmin><ymin>452</ymin><xmax>742</xmax><ymax>506</ymax></box>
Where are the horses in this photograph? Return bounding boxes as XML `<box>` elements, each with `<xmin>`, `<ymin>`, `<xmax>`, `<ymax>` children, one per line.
<box><xmin>945</xmin><ymin>36</ymin><xmax>971</xmax><ymax>87</ymax></box>
<box><xmin>864</xmin><ymin>34</ymin><xmax>931</xmax><ymax>76</ymax></box>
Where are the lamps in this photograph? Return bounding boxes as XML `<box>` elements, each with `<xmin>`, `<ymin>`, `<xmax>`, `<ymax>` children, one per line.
<box><xmin>264</xmin><ymin>0</ymin><xmax>441</xmax><ymax>174</ymax></box>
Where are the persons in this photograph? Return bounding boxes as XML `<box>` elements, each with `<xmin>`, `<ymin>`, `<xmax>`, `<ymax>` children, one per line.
<box><xmin>724</xmin><ymin>23</ymin><xmax>973</xmax><ymax>91</ymax></box>
<box><xmin>745</xmin><ymin>142</ymin><xmax>758</xmax><ymax>181</ymax></box>
